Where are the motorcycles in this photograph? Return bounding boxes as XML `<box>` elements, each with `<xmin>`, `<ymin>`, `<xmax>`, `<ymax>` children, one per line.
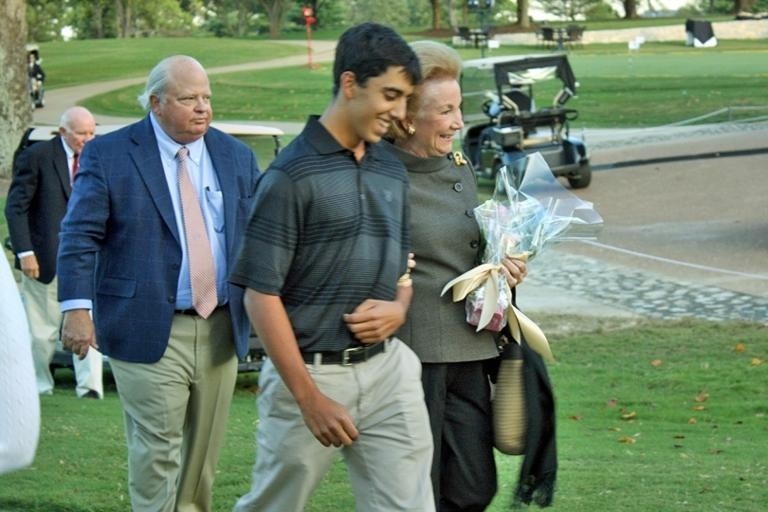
<box><xmin>29</xmin><ymin>68</ymin><xmax>45</xmax><ymax>111</ymax></box>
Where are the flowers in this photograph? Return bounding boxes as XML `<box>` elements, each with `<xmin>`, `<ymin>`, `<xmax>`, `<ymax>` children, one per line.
<box><xmin>466</xmin><ymin>193</ymin><xmax>550</xmax><ymax>329</ymax></box>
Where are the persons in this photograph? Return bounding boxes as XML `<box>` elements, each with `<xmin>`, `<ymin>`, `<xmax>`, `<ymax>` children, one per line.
<box><xmin>394</xmin><ymin>35</ymin><xmax>532</xmax><ymax>511</ymax></box>
<box><xmin>3</xmin><ymin>106</ymin><xmax>105</xmax><ymax>401</ymax></box>
<box><xmin>53</xmin><ymin>51</ymin><xmax>268</xmax><ymax>510</ymax></box>
<box><xmin>223</xmin><ymin>20</ymin><xmax>443</xmax><ymax>512</ymax></box>
<box><xmin>27</xmin><ymin>50</ymin><xmax>45</xmax><ymax>107</ymax></box>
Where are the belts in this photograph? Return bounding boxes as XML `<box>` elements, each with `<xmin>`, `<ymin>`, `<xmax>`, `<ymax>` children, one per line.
<box><xmin>297</xmin><ymin>336</ymin><xmax>393</xmax><ymax>367</ymax></box>
<box><xmin>173</xmin><ymin>309</ymin><xmax>199</xmax><ymax>316</ymax></box>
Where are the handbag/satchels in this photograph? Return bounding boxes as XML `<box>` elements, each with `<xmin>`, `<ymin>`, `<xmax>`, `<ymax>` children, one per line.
<box><xmin>490</xmin><ymin>340</ymin><xmax>549</xmax><ymax>456</ymax></box>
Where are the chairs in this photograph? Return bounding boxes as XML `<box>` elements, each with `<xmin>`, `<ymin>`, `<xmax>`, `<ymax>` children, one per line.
<box><xmin>505</xmin><ymin>83</ymin><xmax>533</xmax><ymax>112</ymax></box>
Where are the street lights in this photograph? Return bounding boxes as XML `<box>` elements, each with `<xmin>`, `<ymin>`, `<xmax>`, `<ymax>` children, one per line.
<box><xmin>467</xmin><ymin>0</ymin><xmax>495</xmax><ymax>58</ymax></box>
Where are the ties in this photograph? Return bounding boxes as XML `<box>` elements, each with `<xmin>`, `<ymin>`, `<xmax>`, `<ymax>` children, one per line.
<box><xmin>73</xmin><ymin>153</ymin><xmax>78</xmax><ymax>183</ymax></box>
<box><xmin>173</xmin><ymin>146</ymin><xmax>221</xmax><ymax>322</ymax></box>
